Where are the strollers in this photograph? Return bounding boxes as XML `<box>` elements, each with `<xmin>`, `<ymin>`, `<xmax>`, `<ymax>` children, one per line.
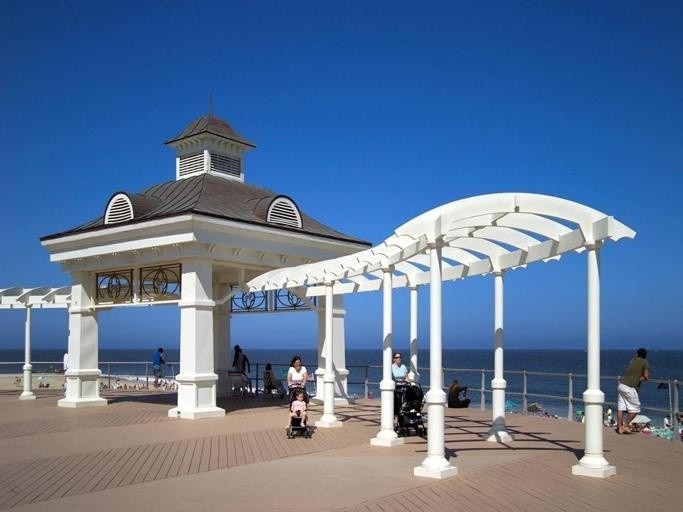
<box><xmin>285</xmin><ymin>387</ymin><xmax>311</xmax><ymax>439</ymax></box>
<box><xmin>393</xmin><ymin>380</ymin><xmax>426</xmax><ymax>438</ymax></box>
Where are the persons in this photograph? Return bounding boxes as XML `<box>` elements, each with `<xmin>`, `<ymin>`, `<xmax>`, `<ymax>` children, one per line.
<box><xmin>231</xmin><ymin>344</ymin><xmax>244</xmax><ymax>390</ymax></box>
<box><xmin>240</xmin><ymin>348</ymin><xmax>250</xmax><ymax>395</ymax></box>
<box><xmin>262</xmin><ymin>362</ymin><xmax>281</xmax><ymax>394</ymax></box>
<box><xmin>99</xmin><ymin>376</ymin><xmax>178</xmax><ymax>390</ymax></box>
<box><xmin>581</xmin><ymin>412</ymin><xmax>585</xmax><ymax>423</ymax></box>
<box><xmin>679</xmin><ymin>415</ymin><xmax>683</xmax><ymax>433</ymax></box>
<box><xmin>616</xmin><ymin>347</ymin><xmax>649</xmax><ymax>434</ymax></box>
<box><xmin>447</xmin><ymin>379</ymin><xmax>471</xmax><ymax>408</ymax></box>
<box><xmin>286</xmin><ymin>355</ymin><xmax>308</xmax><ymax>391</ymax></box>
<box><xmin>604</xmin><ymin>405</ymin><xmax>612</xmax><ymax>423</ymax></box>
<box><xmin>285</xmin><ymin>391</ymin><xmax>308</xmax><ymax>429</ymax></box>
<box><xmin>13</xmin><ymin>375</ymin><xmax>49</xmax><ymax>388</ymax></box>
<box><xmin>150</xmin><ymin>347</ymin><xmax>168</xmax><ymax>383</ymax></box>
<box><xmin>663</xmin><ymin>416</ymin><xmax>672</xmax><ymax>428</ymax></box>
<box><xmin>392</xmin><ymin>352</ymin><xmax>410</xmax><ymax>432</ymax></box>
<box><xmin>544</xmin><ymin>411</ymin><xmax>558</xmax><ymax>419</ymax></box>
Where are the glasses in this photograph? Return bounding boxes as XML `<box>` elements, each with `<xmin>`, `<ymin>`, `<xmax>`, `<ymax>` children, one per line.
<box><xmin>394</xmin><ymin>357</ymin><xmax>401</xmax><ymax>358</ymax></box>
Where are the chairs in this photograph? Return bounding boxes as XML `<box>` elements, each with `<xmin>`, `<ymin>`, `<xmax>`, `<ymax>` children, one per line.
<box><xmin>262</xmin><ymin>370</ymin><xmax>279</xmax><ymax>394</ymax></box>
<box><xmin>228</xmin><ymin>374</ymin><xmax>249</xmax><ymax>400</ymax></box>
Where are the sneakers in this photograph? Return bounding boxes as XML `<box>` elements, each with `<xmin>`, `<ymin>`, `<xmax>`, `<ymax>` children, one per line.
<box><xmin>285</xmin><ymin>425</ymin><xmax>291</xmax><ymax>429</ymax></box>
<box><xmin>301</xmin><ymin>423</ymin><xmax>305</xmax><ymax>427</ymax></box>
